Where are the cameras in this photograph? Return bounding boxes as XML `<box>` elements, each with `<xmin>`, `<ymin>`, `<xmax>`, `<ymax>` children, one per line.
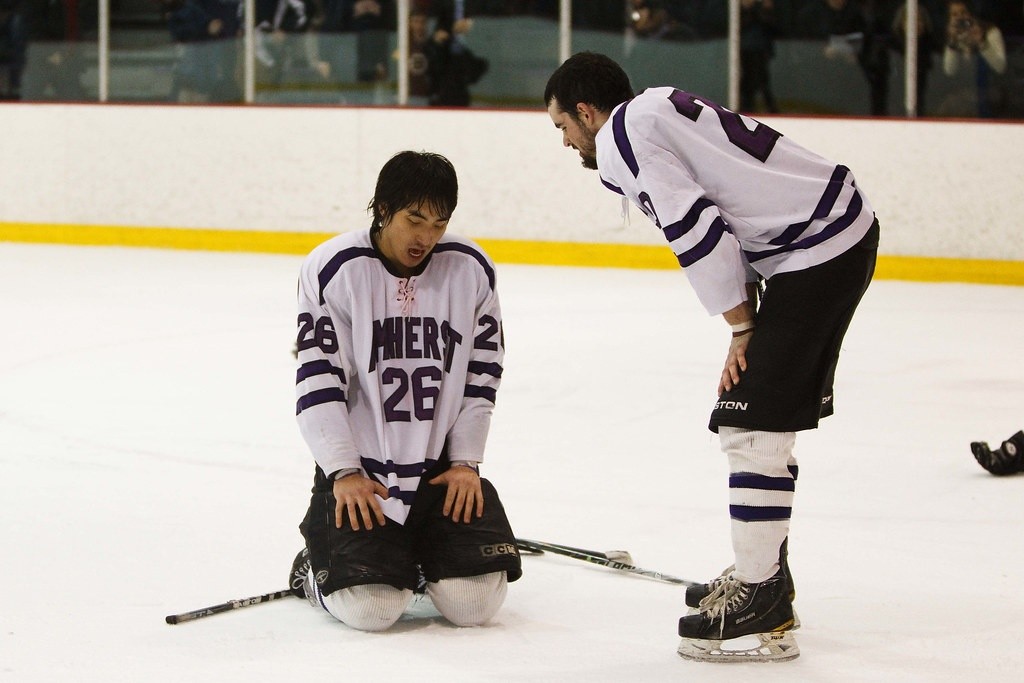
<box><xmin>955</xmin><ymin>20</ymin><xmax>972</xmax><ymax>30</ymax></box>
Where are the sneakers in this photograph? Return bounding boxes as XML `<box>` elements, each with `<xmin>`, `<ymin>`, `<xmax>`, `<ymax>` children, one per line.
<box><xmin>289</xmin><ymin>547</ymin><xmax>312</xmax><ymax>599</ymax></box>
<box><xmin>676</xmin><ymin>573</ymin><xmax>798</xmax><ymax>662</ymax></box>
<box><xmin>685</xmin><ymin>536</ymin><xmax>796</xmax><ymax>618</ymax></box>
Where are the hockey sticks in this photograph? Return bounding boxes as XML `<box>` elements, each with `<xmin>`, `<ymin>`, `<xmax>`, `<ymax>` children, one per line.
<box><xmin>514</xmin><ymin>539</ymin><xmax>703</xmax><ymax>586</ymax></box>
<box><xmin>166</xmin><ymin>550</ymin><xmax>632</xmax><ymax>624</ymax></box>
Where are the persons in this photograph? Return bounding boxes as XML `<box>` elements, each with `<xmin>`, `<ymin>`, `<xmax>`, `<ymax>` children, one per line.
<box><xmin>543</xmin><ymin>51</ymin><xmax>880</xmax><ymax>664</ymax></box>
<box><xmin>288</xmin><ymin>149</ymin><xmax>523</xmax><ymax>632</ymax></box>
<box><xmin>158</xmin><ymin>0</ymin><xmax>1009</xmax><ymax>119</ymax></box>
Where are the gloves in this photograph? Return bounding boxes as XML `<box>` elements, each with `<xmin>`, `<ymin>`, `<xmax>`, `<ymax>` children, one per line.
<box><xmin>970</xmin><ymin>430</ymin><xmax>1024</xmax><ymax>476</ymax></box>
